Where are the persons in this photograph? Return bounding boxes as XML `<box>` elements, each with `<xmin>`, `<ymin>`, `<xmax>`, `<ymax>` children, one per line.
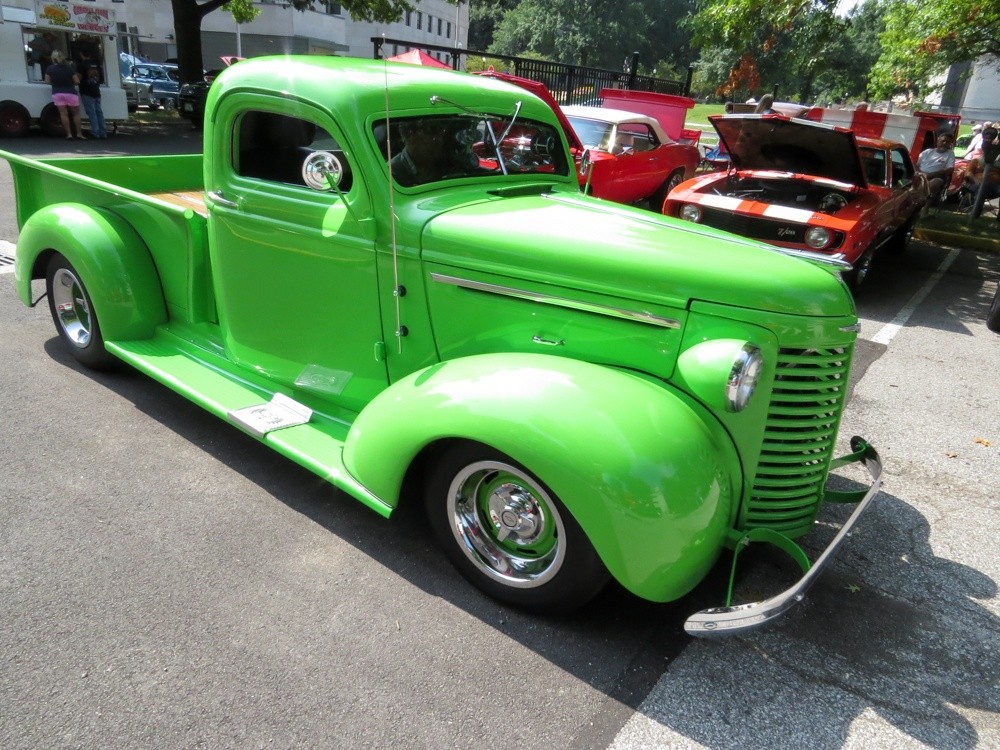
<box><xmin>915</xmin><ymin>134</ymin><xmax>955</xmax><ymax>220</ymax></box>
<box><xmin>231</xmin><ymin>57</ymin><xmax>237</xmax><ymax>66</ymax></box>
<box><xmin>27</xmin><ymin>31</ymin><xmax>53</xmax><ymax>79</ymax></box>
<box><xmin>949</xmin><ymin>122</ymin><xmax>1000</xmax><ymax>209</ymax></box>
<box><xmin>386</xmin><ymin>121</ymin><xmax>466</xmax><ymax>188</ymax></box>
<box><xmin>724</xmin><ymin>94</ymin><xmax>870</xmax><ymax>114</ymax></box>
<box><xmin>45</xmin><ymin>45</ymin><xmax>108</xmax><ymax>139</ymax></box>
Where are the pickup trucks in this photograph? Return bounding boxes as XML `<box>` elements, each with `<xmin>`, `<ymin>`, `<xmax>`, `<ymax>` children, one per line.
<box><xmin>0</xmin><ymin>56</ymin><xmax>886</xmax><ymax>642</ymax></box>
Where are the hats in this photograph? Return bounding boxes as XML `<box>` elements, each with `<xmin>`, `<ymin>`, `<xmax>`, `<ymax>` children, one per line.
<box><xmin>971</xmin><ymin>124</ymin><xmax>982</xmax><ymax>131</ymax></box>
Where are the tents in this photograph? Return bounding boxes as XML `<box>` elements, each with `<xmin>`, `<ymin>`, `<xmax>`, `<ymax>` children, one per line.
<box><xmin>383</xmin><ymin>50</ymin><xmax>453</xmax><ymax>69</ymax></box>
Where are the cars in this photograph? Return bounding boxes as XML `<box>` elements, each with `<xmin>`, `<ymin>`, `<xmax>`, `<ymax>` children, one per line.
<box><xmin>178</xmin><ymin>81</ymin><xmax>210</xmax><ymax>132</ymax></box>
<box><xmin>468</xmin><ymin>65</ymin><xmax>701</xmax><ymax>215</ymax></box>
<box><xmin>662</xmin><ymin>101</ymin><xmax>962</xmax><ymax>297</ymax></box>
<box><xmin>119</xmin><ymin>52</ymin><xmax>246</xmax><ymax>112</ymax></box>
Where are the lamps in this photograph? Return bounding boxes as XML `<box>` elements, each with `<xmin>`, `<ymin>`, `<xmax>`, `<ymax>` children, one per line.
<box><xmin>165</xmin><ymin>34</ymin><xmax>174</xmax><ymax>39</ymax></box>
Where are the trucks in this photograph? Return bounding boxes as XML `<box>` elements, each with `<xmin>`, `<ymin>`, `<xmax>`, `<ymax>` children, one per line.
<box><xmin>0</xmin><ymin>0</ymin><xmax>130</xmax><ymax>139</ymax></box>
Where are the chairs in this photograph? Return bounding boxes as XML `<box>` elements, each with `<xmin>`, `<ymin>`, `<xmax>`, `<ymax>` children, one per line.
<box><xmin>864</xmin><ymin>158</ymin><xmax>886</xmax><ymax>184</ymax></box>
<box><xmin>615</xmin><ymin>133</ymin><xmax>634</xmax><ymax>151</ymax></box>
<box><xmin>245</xmin><ymin>112</ymin><xmax>462</xmax><ymax>190</ymax></box>
<box><xmin>934</xmin><ymin>163</ymin><xmax>1000</xmax><ymax>221</ymax></box>
<box><xmin>678</xmin><ymin>129</ymin><xmax>730</xmax><ymax>174</ymax></box>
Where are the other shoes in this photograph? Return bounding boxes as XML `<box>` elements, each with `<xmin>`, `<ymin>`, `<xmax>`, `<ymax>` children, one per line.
<box><xmin>66</xmin><ymin>134</ymin><xmax>87</xmax><ymax>140</ymax></box>
<box><xmin>88</xmin><ymin>134</ymin><xmax>108</xmax><ymax>139</ymax></box>
